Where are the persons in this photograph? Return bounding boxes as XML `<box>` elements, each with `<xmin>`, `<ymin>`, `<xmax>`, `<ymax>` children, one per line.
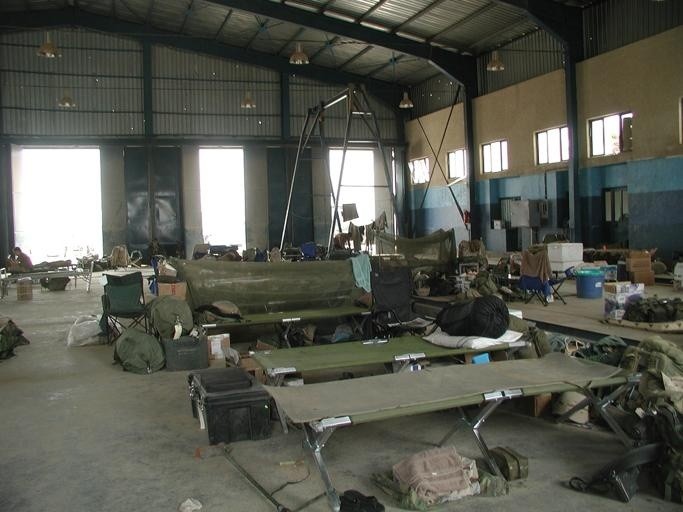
<box><xmin>11</xmin><ymin>247</ymin><xmax>33</xmax><ymax>272</ymax></box>
<box><xmin>334</xmin><ymin>233</ymin><xmax>352</xmax><ymax>251</ymax></box>
<box><xmin>147</xmin><ymin>238</ymin><xmax>168</xmax><ymax>277</ymax></box>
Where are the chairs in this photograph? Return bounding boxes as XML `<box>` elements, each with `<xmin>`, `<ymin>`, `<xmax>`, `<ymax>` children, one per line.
<box><xmin>100</xmin><ymin>272</ymin><xmax>150</xmax><ymax>345</ymax></box>
<box><xmin>459</xmin><ymin>241</ymin><xmax>482</xmax><ymax>276</ymax></box>
<box><xmin>521</xmin><ymin>249</ymin><xmax>567</xmax><ymax>306</ymax></box>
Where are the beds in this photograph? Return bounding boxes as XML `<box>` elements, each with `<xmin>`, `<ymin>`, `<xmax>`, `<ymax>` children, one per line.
<box><xmin>0</xmin><ymin>259</ymin><xmax>97</xmax><ymax>296</ymax></box>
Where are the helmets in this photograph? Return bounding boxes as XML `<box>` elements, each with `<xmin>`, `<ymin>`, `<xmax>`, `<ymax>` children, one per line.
<box><xmin>551</xmin><ymin>391</ymin><xmax>591</xmax><ymax>424</ymax></box>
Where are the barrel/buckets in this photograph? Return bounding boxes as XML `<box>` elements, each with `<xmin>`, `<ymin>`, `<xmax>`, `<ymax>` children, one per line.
<box><xmin>575</xmin><ymin>271</ymin><xmax>605</xmax><ymax>298</ymax></box>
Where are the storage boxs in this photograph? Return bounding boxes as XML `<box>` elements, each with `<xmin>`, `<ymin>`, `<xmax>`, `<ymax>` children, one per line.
<box><xmin>626</xmin><ymin>248</ymin><xmax>654</xmax><ymax>286</ymax></box>
<box><xmin>547</xmin><ymin>242</ymin><xmax>583</xmax><ymax>263</ymax></box>
<box><xmin>157</xmin><ymin>281</ymin><xmax>188</xmax><ymax>300</ymax></box>
<box><xmin>547</xmin><ymin>262</ymin><xmax>584</xmax><ymax>278</ymax></box>
<box><xmin>163</xmin><ymin>336</ymin><xmax>209</xmax><ymax>370</ymax></box>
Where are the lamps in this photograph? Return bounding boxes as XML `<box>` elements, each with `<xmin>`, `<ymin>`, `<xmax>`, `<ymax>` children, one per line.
<box><xmin>240</xmin><ymin>91</ymin><xmax>257</xmax><ymax>109</ymax></box>
<box><xmin>289</xmin><ymin>42</ymin><xmax>310</xmax><ymax>64</ymax></box>
<box><xmin>56</xmin><ymin>90</ymin><xmax>78</xmax><ymax>107</ymax></box>
<box><xmin>399</xmin><ymin>93</ymin><xmax>413</xmax><ymax>108</ymax></box>
<box><xmin>486</xmin><ymin>50</ymin><xmax>505</xmax><ymax>71</ymax></box>
<box><xmin>33</xmin><ymin>32</ymin><xmax>62</xmax><ymax>58</ymax></box>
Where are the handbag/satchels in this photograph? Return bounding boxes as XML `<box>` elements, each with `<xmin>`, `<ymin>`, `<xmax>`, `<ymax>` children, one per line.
<box><xmin>428</xmin><ymin>278</ymin><xmax>459</xmax><ymax>296</ymax></box>
<box><xmin>435</xmin><ymin>295</ymin><xmax>509</xmax><ymax>339</ymax></box>
<box><xmin>469</xmin><ymin>271</ymin><xmax>498</xmax><ymax>296</ymax></box>
<box><xmin>624</xmin><ymin>293</ymin><xmax>682</xmax><ymax>323</ymax></box>
